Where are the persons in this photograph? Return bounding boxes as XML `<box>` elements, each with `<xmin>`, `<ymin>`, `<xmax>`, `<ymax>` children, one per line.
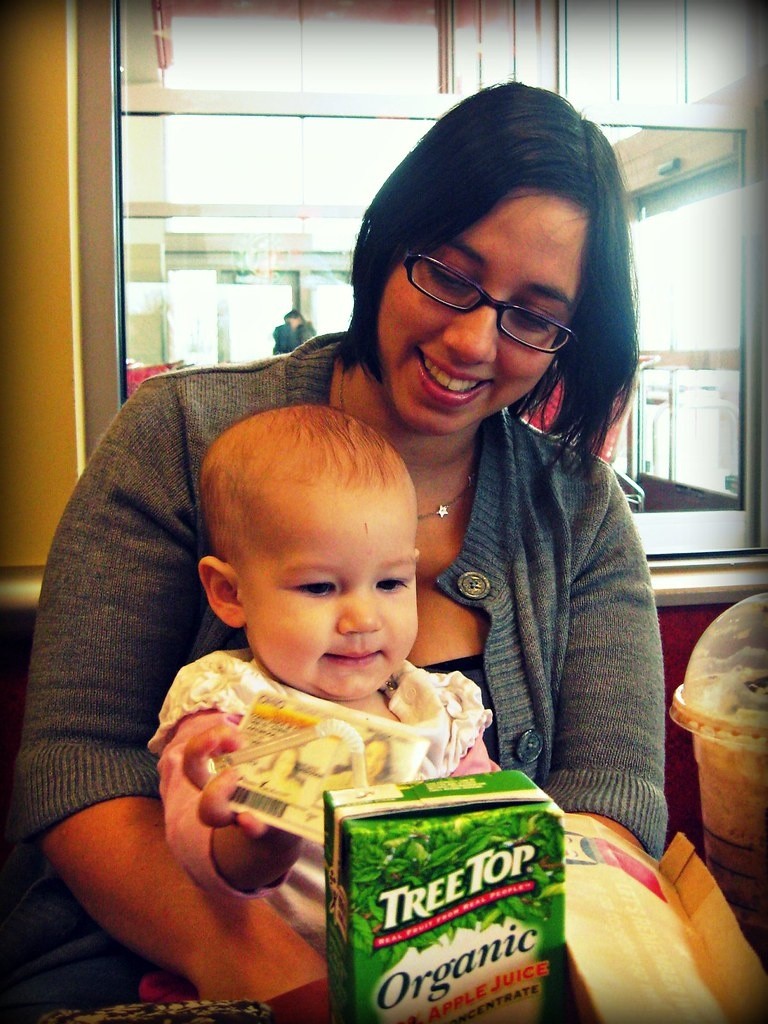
<box><xmin>147</xmin><ymin>404</ymin><xmax>501</xmax><ymax>959</ymax></box>
<box><xmin>259</xmin><ymin>744</ymin><xmax>304</xmax><ymax>793</ymax></box>
<box><xmin>330</xmin><ymin>734</ymin><xmax>392</xmax><ymax>789</ymax></box>
<box><xmin>14</xmin><ymin>84</ymin><xmax>667</xmax><ymax>1002</ymax></box>
<box><xmin>273</xmin><ymin>311</ymin><xmax>316</xmax><ymax>354</ymax></box>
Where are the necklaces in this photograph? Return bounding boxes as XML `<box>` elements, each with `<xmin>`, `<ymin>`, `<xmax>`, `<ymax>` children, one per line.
<box><xmin>339</xmin><ymin>361</ymin><xmax>473</xmax><ymax>519</ymax></box>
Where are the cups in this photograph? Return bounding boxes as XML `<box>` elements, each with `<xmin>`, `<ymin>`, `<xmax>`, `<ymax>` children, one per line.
<box><xmin>668</xmin><ymin>683</ymin><xmax>768</xmax><ymax>928</ymax></box>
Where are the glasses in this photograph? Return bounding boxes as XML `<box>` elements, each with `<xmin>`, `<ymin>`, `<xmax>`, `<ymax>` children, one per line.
<box><xmin>400</xmin><ymin>237</ymin><xmax>577</xmax><ymax>354</ymax></box>
